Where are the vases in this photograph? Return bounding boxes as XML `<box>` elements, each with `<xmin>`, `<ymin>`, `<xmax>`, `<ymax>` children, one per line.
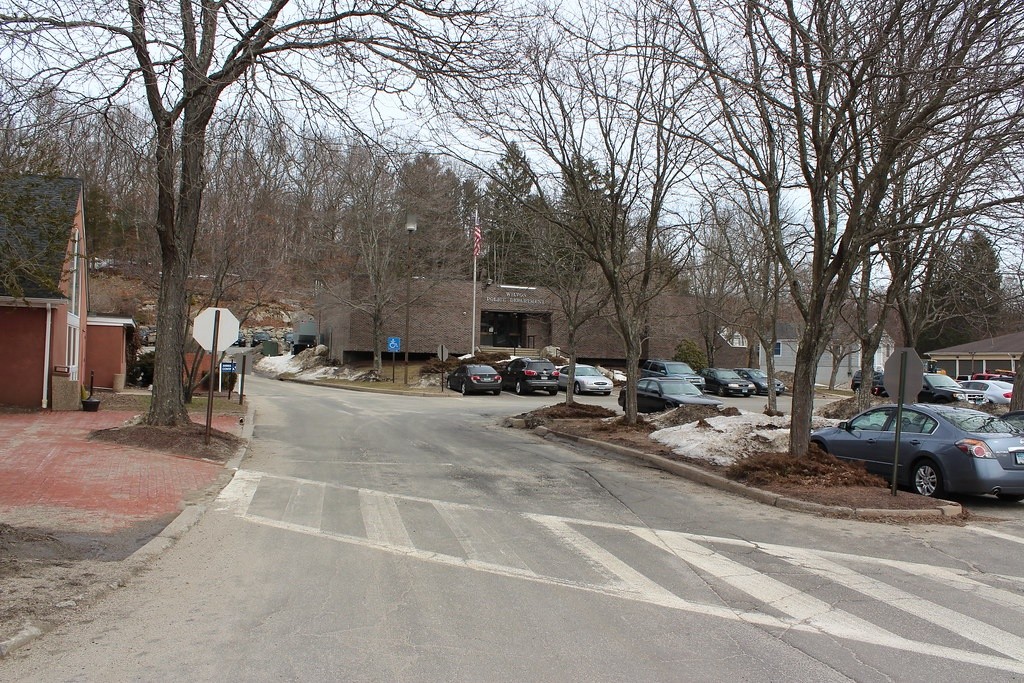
<box><xmin>82</xmin><ymin>401</ymin><xmax>100</xmax><ymax>412</ymax></box>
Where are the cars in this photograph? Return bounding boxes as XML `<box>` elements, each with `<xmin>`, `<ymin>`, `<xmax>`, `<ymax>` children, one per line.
<box><xmin>734</xmin><ymin>368</ymin><xmax>783</xmax><ymax>396</ymax></box>
<box><xmin>952</xmin><ymin>373</ymin><xmax>1014</xmax><ymax>403</ymax></box>
<box><xmin>281</xmin><ymin>332</ymin><xmax>294</xmax><ymax>348</ymax></box>
<box><xmin>447</xmin><ymin>364</ymin><xmax>502</xmax><ymax>394</ymax></box>
<box><xmin>138</xmin><ymin>330</ymin><xmax>149</xmax><ymax>347</ymax></box>
<box><xmin>695</xmin><ymin>368</ymin><xmax>756</xmax><ymax>397</ymax></box>
<box><xmin>812</xmin><ymin>401</ymin><xmax>1024</xmax><ymax>501</ymax></box>
<box><xmin>618</xmin><ymin>376</ymin><xmax>726</xmax><ymax>413</ymax></box>
<box><xmin>557</xmin><ymin>364</ymin><xmax>614</xmax><ymax>395</ymax></box>
<box><xmin>250</xmin><ymin>331</ymin><xmax>274</xmax><ymax>347</ymax></box>
<box><xmin>229</xmin><ymin>331</ymin><xmax>247</xmax><ymax>347</ymax></box>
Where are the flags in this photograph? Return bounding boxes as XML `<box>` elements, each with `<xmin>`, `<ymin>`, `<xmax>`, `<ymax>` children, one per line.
<box><xmin>474</xmin><ymin>209</ymin><xmax>481</xmax><ymax>257</ymax></box>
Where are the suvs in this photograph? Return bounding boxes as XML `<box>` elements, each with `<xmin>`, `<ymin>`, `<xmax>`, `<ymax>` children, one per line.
<box><xmin>500</xmin><ymin>357</ymin><xmax>560</xmax><ymax>395</ymax></box>
<box><xmin>639</xmin><ymin>360</ymin><xmax>706</xmax><ymax>394</ymax></box>
<box><xmin>851</xmin><ymin>368</ymin><xmax>884</xmax><ymax>394</ymax></box>
<box><xmin>874</xmin><ymin>372</ymin><xmax>989</xmax><ymax>406</ymax></box>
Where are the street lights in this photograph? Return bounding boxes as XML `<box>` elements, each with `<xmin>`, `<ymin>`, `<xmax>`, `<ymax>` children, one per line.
<box><xmin>404</xmin><ymin>212</ymin><xmax>417</xmax><ymax>384</ymax></box>
